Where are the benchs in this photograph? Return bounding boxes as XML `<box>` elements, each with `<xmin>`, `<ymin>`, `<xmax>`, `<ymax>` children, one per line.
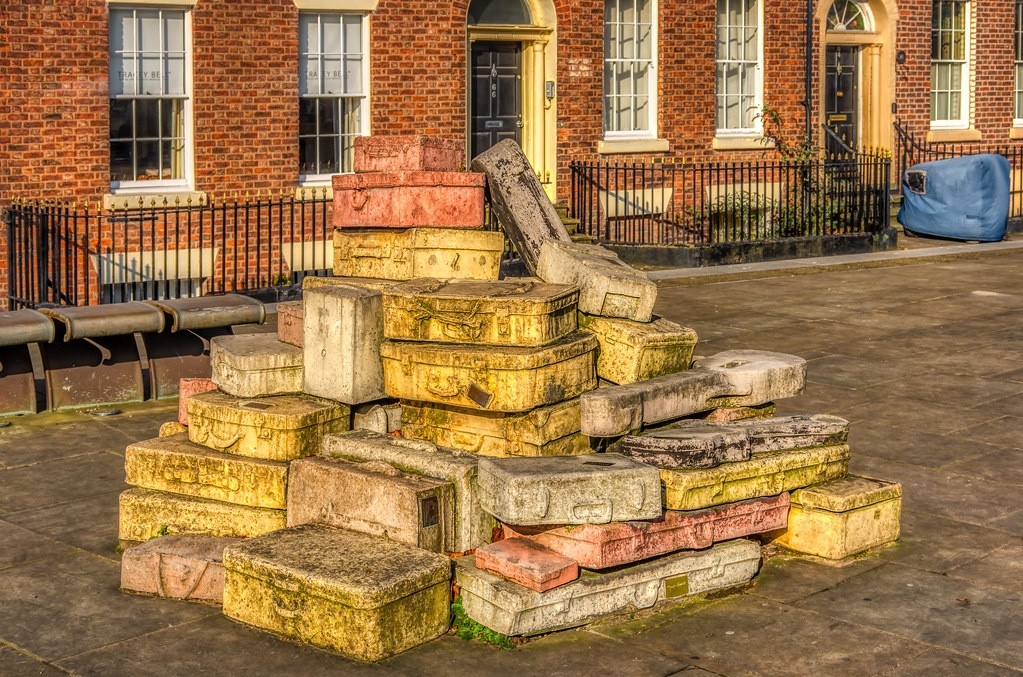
<box><xmin>0</xmin><ymin>292</ymin><xmax>266</xmax><ymax>416</ymax></box>
